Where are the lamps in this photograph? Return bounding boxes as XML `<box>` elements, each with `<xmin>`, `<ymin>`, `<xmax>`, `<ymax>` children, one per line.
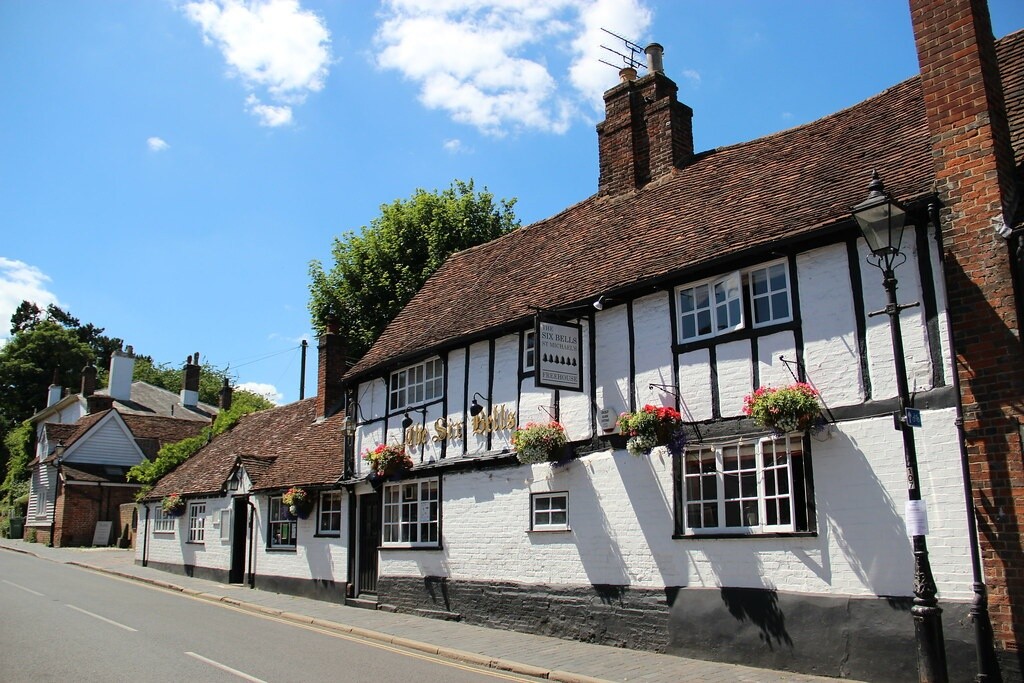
<box><xmin>593</xmin><ymin>295</ymin><xmax>612</xmax><ymax>310</ymax></box>
<box><xmin>470</xmin><ymin>392</ymin><xmax>488</xmax><ymax>416</ymax></box>
<box><xmin>402</xmin><ymin>406</ymin><xmax>426</xmax><ymax>429</ymax></box>
<box><xmin>340</xmin><ymin>402</ymin><xmax>371</xmax><ymax>436</ymax></box>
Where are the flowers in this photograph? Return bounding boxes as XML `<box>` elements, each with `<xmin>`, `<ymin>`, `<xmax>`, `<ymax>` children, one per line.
<box><xmin>616</xmin><ymin>404</ymin><xmax>686</xmax><ymax>455</ymax></box>
<box><xmin>283</xmin><ymin>486</ymin><xmax>307</xmax><ymax>505</ymax></box>
<box><xmin>510</xmin><ymin>421</ymin><xmax>566</xmax><ymax>463</ymax></box>
<box><xmin>742</xmin><ymin>382</ymin><xmax>828</xmax><ymax>438</ymax></box>
<box><xmin>360</xmin><ymin>441</ymin><xmax>413</xmax><ymax>477</ymax></box>
<box><xmin>162</xmin><ymin>488</ymin><xmax>184</xmax><ymax>513</ymax></box>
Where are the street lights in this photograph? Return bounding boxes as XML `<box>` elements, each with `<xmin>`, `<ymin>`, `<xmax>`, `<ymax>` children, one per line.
<box><xmin>48</xmin><ymin>438</ymin><xmax>67</xmax><ymax>547</ymax></box>
<box><xmin>850</xmin><ymin>168</ymin><xmax>948</xmax><ymax>683</ymax></box>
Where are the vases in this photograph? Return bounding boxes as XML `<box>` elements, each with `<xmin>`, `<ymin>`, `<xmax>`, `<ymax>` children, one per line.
<box><xmin>169</xmin><ymin>507</ymin><xmax>180</xmax><ymax>516</ymax></box>
<box><xmin>797</xmin><ymin>416</ymin><xmax>809</xmax><ymax>430</ymax></box>
<box><xmin>290</xmin><ymin>505</ymin><xmax>301</xmax><ymax>515</ymax></box>
<box><xmin>387</xmin><ymin>465</ymin><xmax>401</xmax><ymax>480</ymax></box>
<box><xmin>654</xmin><ymin>434</ymin><xmax>666</xmax><ymax>446</ymax></box>
